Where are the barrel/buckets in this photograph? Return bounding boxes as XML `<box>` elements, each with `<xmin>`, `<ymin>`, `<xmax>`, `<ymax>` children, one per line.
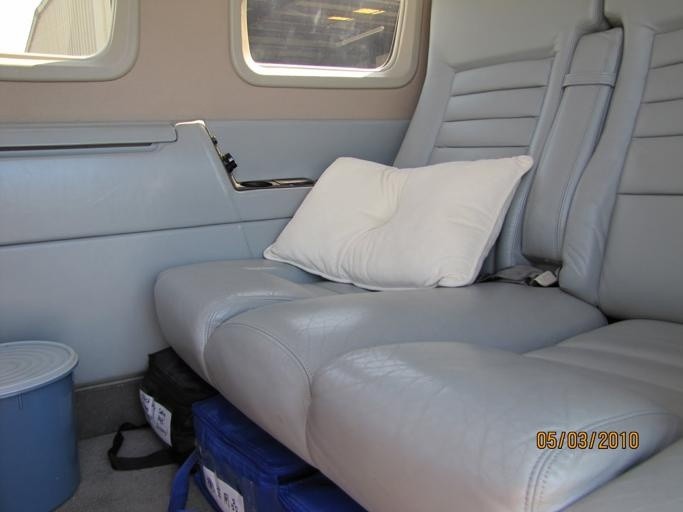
<box><xmin>0</xmin><ymin>341</ymin><xmax>80</xmax><ymax>512</ymax></box>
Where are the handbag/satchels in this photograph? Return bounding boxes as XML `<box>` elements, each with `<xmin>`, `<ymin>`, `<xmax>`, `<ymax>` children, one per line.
<box><xmin>108</xmin><ymin>347</ymin><xmax>223</xmax><ymax>472</ymax></box>
<box><xmin>169</xmin><ymin>392</ymin><xmax>366</xmax><ymax>512</ymax></box>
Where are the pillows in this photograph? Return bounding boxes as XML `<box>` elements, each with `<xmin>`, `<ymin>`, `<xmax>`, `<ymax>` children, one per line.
<box><xmin>262</xmin><ymin>155</ymin><xmax>537</xmax><ymax>292</ymax></box>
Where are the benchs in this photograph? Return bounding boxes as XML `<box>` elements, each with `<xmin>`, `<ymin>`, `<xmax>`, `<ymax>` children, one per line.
<box><xmin>153</xmin><ymin>0</ymin><xmax>683</xmax><ymax>512</ymax></box>
